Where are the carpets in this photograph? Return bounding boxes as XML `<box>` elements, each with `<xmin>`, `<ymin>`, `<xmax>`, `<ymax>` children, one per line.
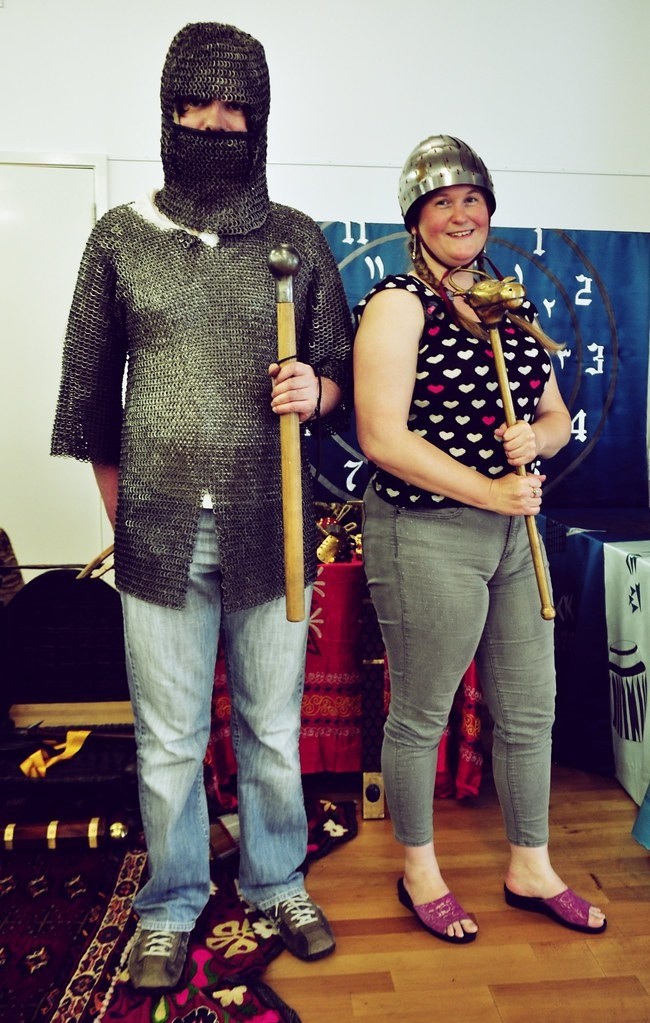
<box><xmin>0</xmin><ymin>793</ymin><xmax>360</xmax><ymax>1023</ymax></box>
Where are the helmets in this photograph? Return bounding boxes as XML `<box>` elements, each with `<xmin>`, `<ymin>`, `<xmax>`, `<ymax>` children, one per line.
<box><xmin>399</xmin><ymin>134</ymin><xmax>497</xmax><ymax>228</ymax></box>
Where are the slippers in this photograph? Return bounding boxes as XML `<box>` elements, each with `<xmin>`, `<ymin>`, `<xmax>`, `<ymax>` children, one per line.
<box><xmin>398</xmin><ymin>876</ymin><xmax>477</xmax><ymax>945</ymax></box>
<box><xmin>504</xmin><ymin>883</ymin><xmax>608</xmax><ymax>934</ymax></box>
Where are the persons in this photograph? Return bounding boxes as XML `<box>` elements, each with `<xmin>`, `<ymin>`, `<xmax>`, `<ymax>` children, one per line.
<box><xmin>52</xmin><ymin>22</ymin><xmax>357</xmax><ymax>989</ymax></box>
<box><xmin>352</xmin><ymin>134</ymin><xmax>608</xmax><ymax>944</ymax></box>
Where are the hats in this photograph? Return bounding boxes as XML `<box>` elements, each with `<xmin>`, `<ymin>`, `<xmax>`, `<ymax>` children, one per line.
<box><xmin>153</xmin><ymin>22</ymin><xmax>271</xmax><ymax>235</ymax></box>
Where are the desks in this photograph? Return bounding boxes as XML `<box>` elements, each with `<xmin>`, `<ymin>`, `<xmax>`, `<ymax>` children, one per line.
<box><xmin>206</xmin><ymin>553</ymin><xmax>483</xmax><ymax>819</ymax></box>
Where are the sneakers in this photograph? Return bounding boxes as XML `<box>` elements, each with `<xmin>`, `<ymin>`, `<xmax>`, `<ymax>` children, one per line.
<box><xmin>267</xmin><ymin>898</ymin><xmax>335</xmax><ymax>960</ymax></box>
<box><xmin>131</xmin><ymin>924</ymin><xmax>191</xmax><ymax>992</ymax></box>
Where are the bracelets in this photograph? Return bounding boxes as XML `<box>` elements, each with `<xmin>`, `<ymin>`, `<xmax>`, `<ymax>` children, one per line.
<box><xmin>316</xmin><ymin>376</ymin><xmax>322</xmax><ymax>421</ymax></box>
